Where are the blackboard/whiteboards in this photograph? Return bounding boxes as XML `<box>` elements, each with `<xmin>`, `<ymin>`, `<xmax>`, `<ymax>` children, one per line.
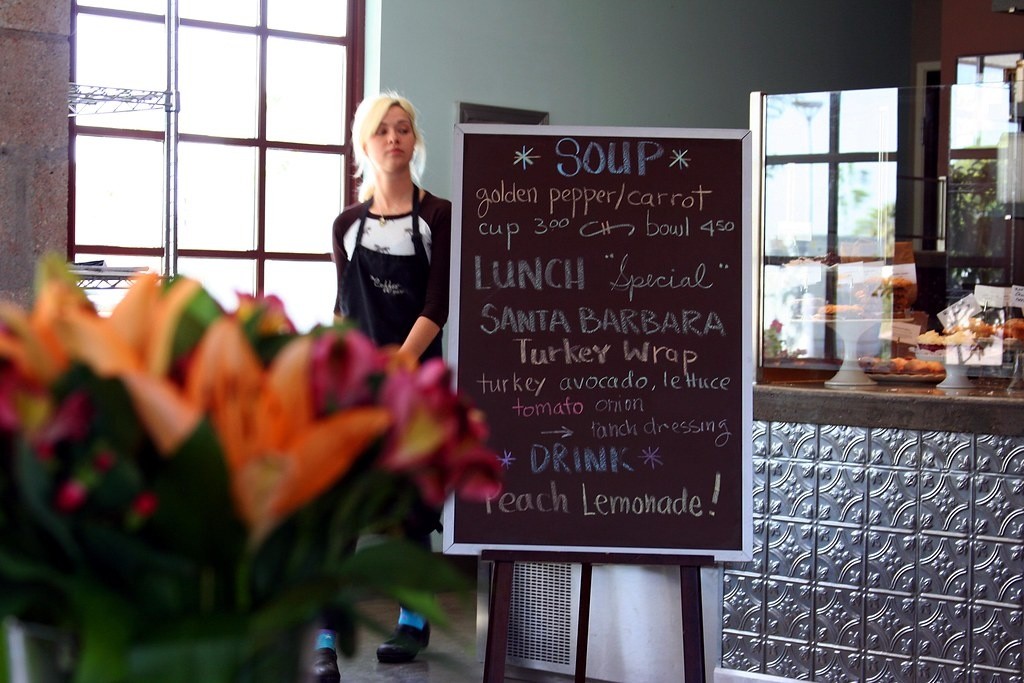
<box><xmin>439</xmin><ymin>122</ymin><xmax>757</xmax><ymax>565</ymax></box>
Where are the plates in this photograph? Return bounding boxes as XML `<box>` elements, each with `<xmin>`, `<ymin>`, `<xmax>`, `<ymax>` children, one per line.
<box><xmin>791</xmin><ymin>317</ymin><xmax>916</xmax><ymax>387</ymax></box>
<box><xmin>866</xmin><ymin>373</ymin><xmax>947</xmax><ymax>385</ymax></box>
<box><xmin>977</xmin><ymin>337</ymin><xmax>1018</xmax><ymax>348</ymax></box>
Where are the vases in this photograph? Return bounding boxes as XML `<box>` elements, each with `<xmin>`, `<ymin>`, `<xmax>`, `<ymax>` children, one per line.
<box><xmin>5</xmin><ymin>616</ymin><xmax>321</xmax><ymax>682</ymax></box>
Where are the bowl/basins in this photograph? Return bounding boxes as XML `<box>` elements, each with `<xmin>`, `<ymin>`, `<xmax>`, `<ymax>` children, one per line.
<box><xmin>762</xmin><ymin>358</ymin><xmax>843</xmax><ymax>386</ymax></box>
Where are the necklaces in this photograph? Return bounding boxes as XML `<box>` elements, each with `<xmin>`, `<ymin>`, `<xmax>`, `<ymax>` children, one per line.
<box><xmin>376</xmin><ymin>202</ymin><xmax>386</xmax><ymax>227</ymax></box>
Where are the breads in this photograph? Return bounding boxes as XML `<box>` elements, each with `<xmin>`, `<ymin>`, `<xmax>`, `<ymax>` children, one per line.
<box><xmin>817</xmin><ymin>305</ymin><xmax>865</xmax><ymax>314</ymax></box>
<box><xmin>857</xmin><ymin>357</ymin><xmax>947</xmax><ymax>375</ymax></box>
<box><xmin>940</xmin><ymin>316</ymin><xmax>1024</xmax><ymax>340</ymax></box>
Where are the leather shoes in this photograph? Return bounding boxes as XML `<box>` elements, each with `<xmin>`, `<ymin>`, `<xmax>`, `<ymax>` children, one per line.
<box><xmin>311</xmin><ymin>648</ymin><xmax>340</xmax><ymax>683</ymax></box>
<box><xmin>377</xmin><ymin>620</ymin><xmax>430</xmax><ymax>663</ymax></box>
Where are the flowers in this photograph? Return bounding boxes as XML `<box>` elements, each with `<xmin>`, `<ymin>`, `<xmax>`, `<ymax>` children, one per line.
<box><xmin>0</xmin><ymin>252</ymin><xmax>503</xmax><ymax>681</ymax></box>
<box><xmin>762</xmin><ymin>319</ymin><xmax>810</xmax><ymax>359</ymax></box>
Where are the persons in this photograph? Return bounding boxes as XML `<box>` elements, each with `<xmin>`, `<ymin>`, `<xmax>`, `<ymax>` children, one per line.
<box><xmin>310</xmin><ymin>93</ymin><xmax>452</xmax><ymax>683</ymax></box>
